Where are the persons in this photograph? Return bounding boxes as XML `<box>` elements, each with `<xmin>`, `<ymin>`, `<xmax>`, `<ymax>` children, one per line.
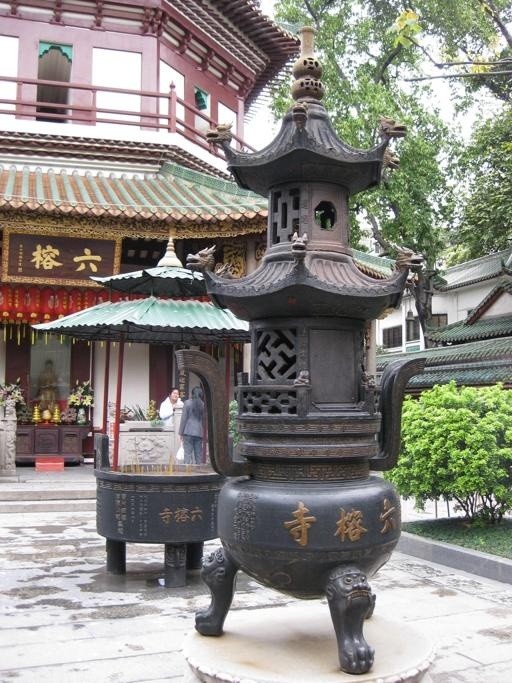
<box><xmin>159</xmin><ymin>387</ymin><xmax>185</xmax><ymax>462</ymax></box>
<box><xmin>37</xmin><ymin>359</ymin><xmax>61</xmax><ymax>411</ymax></box>
<box><xmin>177</xmin><ymin>385</ymin><xmax>206</xmax><ymax>464</ymax></box>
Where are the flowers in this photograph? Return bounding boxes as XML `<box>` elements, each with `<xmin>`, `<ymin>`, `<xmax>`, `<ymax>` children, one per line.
<box><xmin>70</xmin><ymin>380</ymin><xmax>95</xmax><ymax>409</ymax></box>
<box><xmin>61</xmin><ymin>408</ymin><xmax>77</xmax><ymax>425</ymax></box>
<box><xmin>17</xmin><ymin>405</ymin><xmax>32</xmax><ymax>425</ymax></box>
<box><xmin>0</xmin><ymin>377</ymin><xmax>26</xmax><ymax>408</ymax></box>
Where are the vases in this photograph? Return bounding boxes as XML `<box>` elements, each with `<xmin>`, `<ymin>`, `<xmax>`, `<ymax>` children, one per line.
<box><xmin>77</xmin><ymin>407</ymin><xmax>87</xmax><ymax>426</ymax></box>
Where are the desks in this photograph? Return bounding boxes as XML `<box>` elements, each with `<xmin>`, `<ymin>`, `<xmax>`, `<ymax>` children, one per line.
<box><xmin>15</xmin><ymin>422</ymin><xmax>91</xmax><ymax>464</ymax></box>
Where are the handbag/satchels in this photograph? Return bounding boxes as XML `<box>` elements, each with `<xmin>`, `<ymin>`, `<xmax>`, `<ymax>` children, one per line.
<box><xmin>176</xmin><ymin>441</ymin><xmax>184</xmax><ymax>460</ymax></box>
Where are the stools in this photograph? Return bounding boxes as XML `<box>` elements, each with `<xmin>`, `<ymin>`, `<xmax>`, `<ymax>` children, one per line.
<box><xmin>34</xmin><ymin>458</ymin><xmax>65</xmax><ymax>472</ymax></box>
